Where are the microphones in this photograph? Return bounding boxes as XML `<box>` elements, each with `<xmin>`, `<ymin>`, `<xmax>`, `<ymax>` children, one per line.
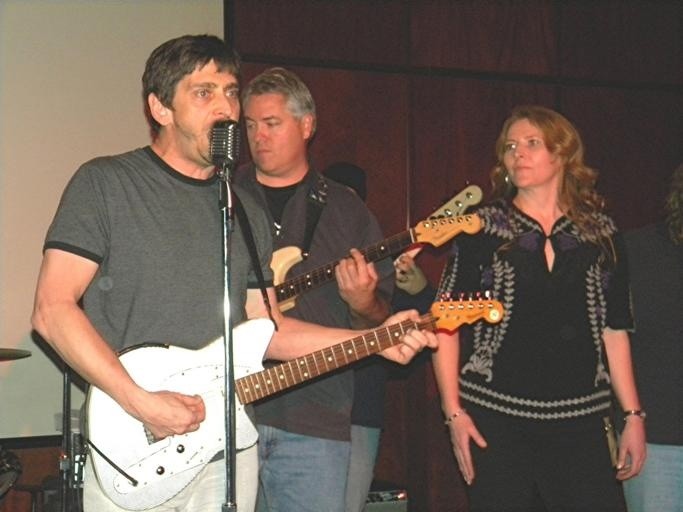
<box><xmin>209</xmin><ymin>120</ymin><xmax>241</xmax><ymax>172</ymax></box>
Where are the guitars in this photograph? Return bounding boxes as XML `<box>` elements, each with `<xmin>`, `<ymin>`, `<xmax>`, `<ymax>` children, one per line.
<box><xmin>270</xmin><ymin>178</ymin><xmax>487</xmax><ymax>312</ymax></box>
<box><xmin>86</xmin><ymin>290</ymin><xmax>503</xmax><ymax>511</ymax></box>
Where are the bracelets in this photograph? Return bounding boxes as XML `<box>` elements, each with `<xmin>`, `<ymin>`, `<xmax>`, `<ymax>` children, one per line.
<box><xmin>444</xmin><ymin>408</ymin><xmax>466</xmax><ymax>424</ymax></box>
<box><xmin>623</xmin><ymin>409</ymin><xmax>646</xmax><ymax>422</ymax></box>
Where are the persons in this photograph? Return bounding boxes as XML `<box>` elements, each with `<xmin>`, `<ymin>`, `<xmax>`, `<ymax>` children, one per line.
<box><xmin>28</xmin><ymin>31</ymin><xmax>437</xmax><ymax>510</ymax></box>
<box><xmin>234</xmin><ymin>65</ymin><xmax>396</xmax><ymax>511</ymax></box>
<box><xmin>427</xmin><ymin>105</ymin><xmax>649</xmax><ymax>511</ymax></box>
<box><xmin>319</xmin><ymin>161</ymin><xmax>435</xmax><ymax>511</ymax></box>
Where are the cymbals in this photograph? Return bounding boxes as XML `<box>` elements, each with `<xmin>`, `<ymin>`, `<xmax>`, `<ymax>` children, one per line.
<box><xmin>0</xmin><ymin>346</ymin><xmax>31</xmax><ymax>362</ymax></box>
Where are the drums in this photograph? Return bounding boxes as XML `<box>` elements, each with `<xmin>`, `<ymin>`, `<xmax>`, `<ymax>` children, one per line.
<box><xmin>0</xmin><ymin>448</ymin><xmax>23</xmax><ymax>499</ymax></box>
